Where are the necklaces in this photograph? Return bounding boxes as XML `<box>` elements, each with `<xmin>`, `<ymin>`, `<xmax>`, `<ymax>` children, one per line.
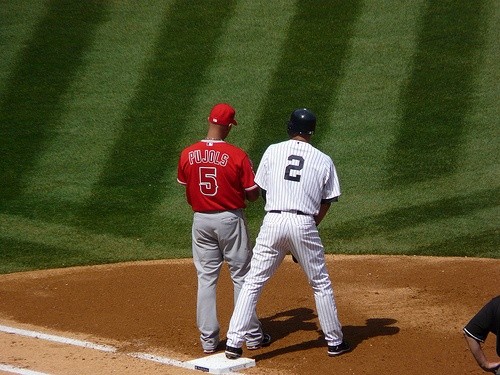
<box><xmin>204</xmin><ymin>138</ymin><xmax>222</xmax><ymax>140</ymax></box>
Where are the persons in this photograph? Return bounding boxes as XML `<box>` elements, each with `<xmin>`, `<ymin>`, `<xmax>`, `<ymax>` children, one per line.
<box><xmin>176</xmin><ymin>103</ymin><xmax>271</xmax><ymax>353</ymax></box>
<box><xmin>225</xmin><ymin>107</ymin><xmax>352</xmax><ymax>358</ymax></box>
<box><xmin>463</xmin><ymin>295</ymin><xmax>500</xmax><ymax>375</ymax></box>
